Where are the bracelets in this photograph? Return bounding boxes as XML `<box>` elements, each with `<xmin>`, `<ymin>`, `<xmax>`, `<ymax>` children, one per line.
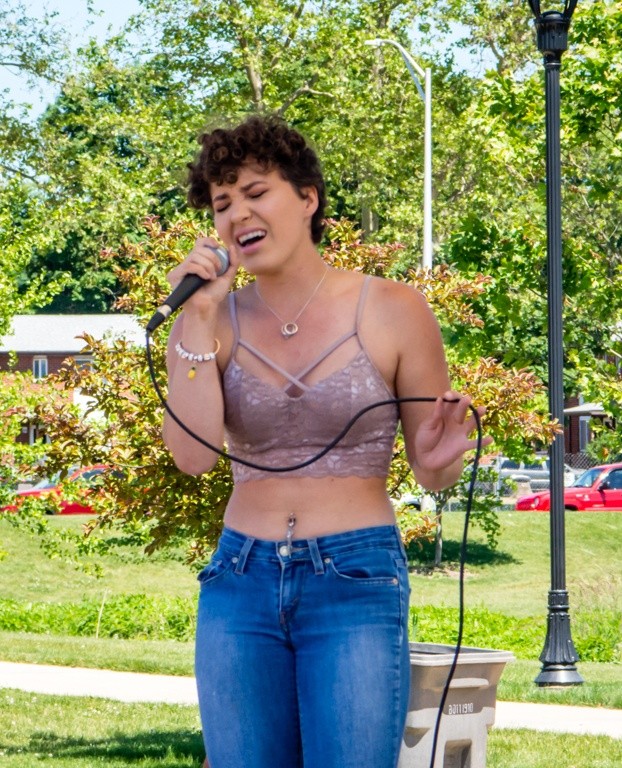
<box><xmin>175</xmin><ymin>337</ymin><xmax>220</xmax><ymax>380</ymax></box>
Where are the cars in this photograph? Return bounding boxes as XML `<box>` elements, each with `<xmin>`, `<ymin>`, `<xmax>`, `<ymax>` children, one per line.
<box><xmin>515</xmin><ymin>462</ymin><xmax>622</xmax><ymax>511</ymax></box>
<box><xmin>0</xmin><ymin>455</ymin><xmax>166</xmax><ymax>518</ymax></box>
<box><xmin>388</xmin><ymin>454</ymin><xmax>592</xmax><ymax>514</ymax></box>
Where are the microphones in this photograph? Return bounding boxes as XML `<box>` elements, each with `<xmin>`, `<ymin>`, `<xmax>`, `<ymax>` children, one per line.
<box><xmin>146</xmin><ymin>248</ymin><xmax>229</xmax><ymax>334</ymax></box>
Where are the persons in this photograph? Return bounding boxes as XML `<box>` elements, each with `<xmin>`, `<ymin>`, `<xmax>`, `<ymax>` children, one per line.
<box><xmin>162</xmin><ymin>115</ymin><xmax>494</xmax><ymax>768</ymax></box>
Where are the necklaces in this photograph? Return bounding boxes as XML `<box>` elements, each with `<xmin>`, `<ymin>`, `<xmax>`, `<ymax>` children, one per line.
<box><xmin>255</xmin><ymin>263</ymin><xmax>328</xmax><ymax>336</ymax></box>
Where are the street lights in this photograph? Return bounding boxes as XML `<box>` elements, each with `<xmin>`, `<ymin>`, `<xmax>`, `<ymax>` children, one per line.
<box><xmin>366</xmin><ymin>37</ymin><xmax>433</xmax><ymax>291</ymax></box>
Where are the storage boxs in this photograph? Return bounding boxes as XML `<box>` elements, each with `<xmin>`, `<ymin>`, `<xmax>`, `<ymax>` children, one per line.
<box><xmin>397</xmin><ymin>643</ymin><xmax>517</xmax><ymax>768</ymax></box>
